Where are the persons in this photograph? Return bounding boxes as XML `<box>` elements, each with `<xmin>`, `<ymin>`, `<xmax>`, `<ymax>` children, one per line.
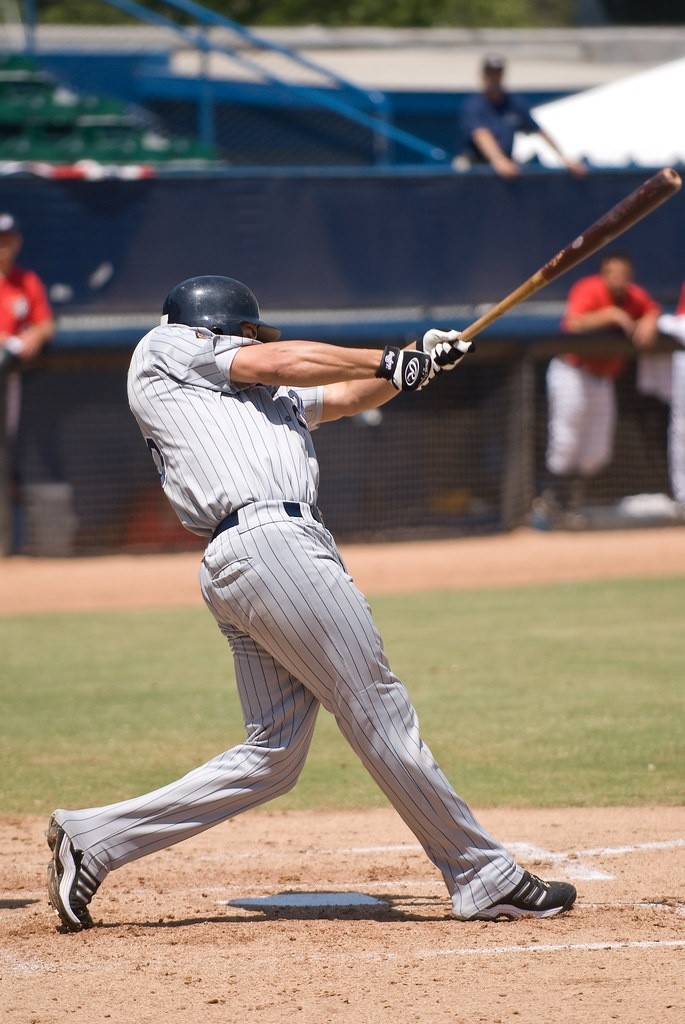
<box><xmin>461</xmin><ymin>56</ymin><xmax>587</xmax><ymax>178</ymax></box>
<box><xmin>0</xmin><ymin>213</ymin><xmax>52</xmax><ymax>557</ymax></box>
<box><xmin>532</xmin><ymin>255</ymin><xmax>662</xmax><ymax>531</ymax></box>
<box><xmin>669</xmin><ymin>285</ymin><xmax>685</xmax><ymax>507</ymax></box>
<box><xmin>47</xmin><ymin>276</ymin><xmax>577</xmax><ymax>931</ymax></box>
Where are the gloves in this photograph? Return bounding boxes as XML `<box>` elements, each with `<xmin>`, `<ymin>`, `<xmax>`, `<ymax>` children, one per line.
<box><xmin>375</xmin><ymin>345</ymin><xmax>444</xmax><ymax>393</ymax></box>
<box><xmin>416</xmin><ymin>326</ymin><xmax>475</xmax><ymax>371</ymax></box>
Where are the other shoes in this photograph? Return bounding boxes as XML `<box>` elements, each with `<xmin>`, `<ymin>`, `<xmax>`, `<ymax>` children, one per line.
<box><xmin>563</xmin><ymin>494</ymin><xmax>588</xmax><ymax>531</ymax></box>
<box><xmin>533</xmin><ymin>489</ymin><xmax>561</xmax><ymax>530</ymax></box>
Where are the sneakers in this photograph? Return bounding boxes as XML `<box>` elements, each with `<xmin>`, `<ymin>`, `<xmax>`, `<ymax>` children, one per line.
<box><xmin>466</xmin><ymin>870</ymin><xmax>577</xmax><ymax>921</ymax></box>
<box><xmin>46</xmin><ymin>808</ymin><xmax>102</xmax><ymax>931</ymax></box>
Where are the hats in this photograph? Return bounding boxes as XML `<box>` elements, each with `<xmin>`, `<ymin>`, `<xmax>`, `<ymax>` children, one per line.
<box><xmin>479</xmin><ymin>52</ymin><xmax>505</xmax><ymax>69</ymax></box>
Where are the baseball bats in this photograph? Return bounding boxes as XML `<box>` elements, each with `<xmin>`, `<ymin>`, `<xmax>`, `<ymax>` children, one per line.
<box><xmin>450</xmin><ymin>165</ymin><xmax>684</xmax><ymax>344</ymax></box>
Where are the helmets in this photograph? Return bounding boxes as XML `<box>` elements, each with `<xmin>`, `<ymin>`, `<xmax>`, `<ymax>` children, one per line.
<box><xmin>162</xmin><ymin>274</ymin><xmax>282</xmax><ymax>345</ymax></box>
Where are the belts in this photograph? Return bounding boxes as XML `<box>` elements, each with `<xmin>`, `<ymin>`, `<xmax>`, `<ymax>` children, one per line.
<box><xmin>209</xmin><ymin>502</ymin><xmax>327</xmax><ymax>544</ymax></box>
